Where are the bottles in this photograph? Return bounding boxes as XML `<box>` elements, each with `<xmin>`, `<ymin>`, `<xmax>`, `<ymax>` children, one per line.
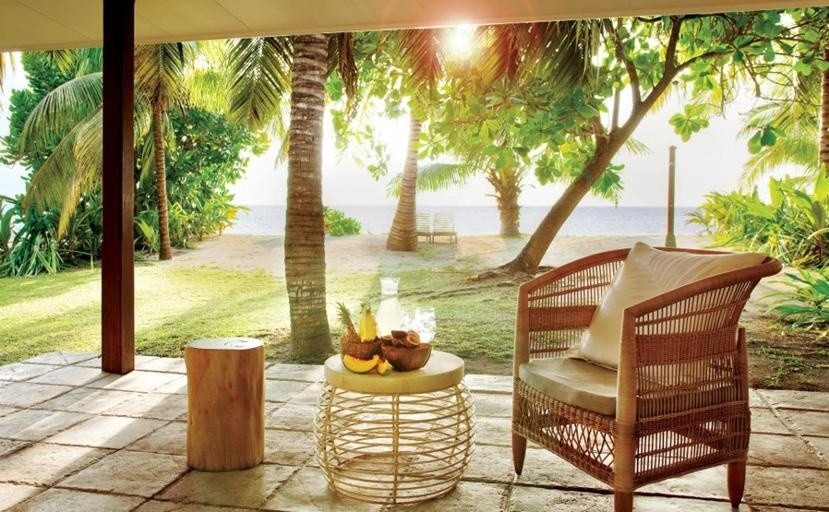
<box><xmin>376</xmin><ymin>277</ymin><xmax>403</xmax><ymax>337</ymax></box>
<box><xmin>357</xmin><ymin>303</ymin><xmax>377</xmax><ymax>341</ymax></box>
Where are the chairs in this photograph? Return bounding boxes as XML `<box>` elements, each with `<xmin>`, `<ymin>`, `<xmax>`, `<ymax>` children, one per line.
<box><xmin>510</xmin><ymin>244</ymin><xmax>785</xmax><ymax>511</ymax></box>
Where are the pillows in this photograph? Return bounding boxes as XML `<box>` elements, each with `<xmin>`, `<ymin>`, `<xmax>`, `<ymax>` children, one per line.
<box><xmin>562</xmin><ymin>240</ymin><xmax>769</xmax><ymax>386</ymax></box>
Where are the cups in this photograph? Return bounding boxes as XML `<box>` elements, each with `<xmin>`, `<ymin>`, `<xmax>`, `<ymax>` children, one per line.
<box><xmin>411</xmin><ymin>307</ymin><xmax>436</xmax><ymax>344</ymax></box>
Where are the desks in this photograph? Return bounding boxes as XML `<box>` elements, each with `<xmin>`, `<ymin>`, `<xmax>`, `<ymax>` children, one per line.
<box><xmin>311</xmin><ymin>353</ymin><xmax>478</xmax><ymax>508</ymax></box>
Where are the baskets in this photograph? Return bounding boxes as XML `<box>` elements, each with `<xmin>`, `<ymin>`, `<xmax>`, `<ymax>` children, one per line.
<box><xmin>337</xmin><ymin>331</ymin><xmax>383</xmax><ymax>375</ymax></box>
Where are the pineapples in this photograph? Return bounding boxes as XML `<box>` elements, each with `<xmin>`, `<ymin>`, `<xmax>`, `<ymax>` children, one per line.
<box><xmin>335</xmin><ymin>301</ymin><xmax>360</xmax><ymax>343</ymax></box>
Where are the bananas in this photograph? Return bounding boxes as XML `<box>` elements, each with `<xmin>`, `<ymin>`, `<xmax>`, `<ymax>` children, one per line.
<box><xmin>360</xmin><ymin>309</ymin><xmax>377</xmax><ymax>343</ymax></box>
<box><xmin>343</xmin><ymin>354</ymin><xmax>380</xmax><ymax>373</ymax></box>
<box><xmin>376</xmin><ymin>358</ymin><xmax>393</xmax><ymax>375</ymax></box>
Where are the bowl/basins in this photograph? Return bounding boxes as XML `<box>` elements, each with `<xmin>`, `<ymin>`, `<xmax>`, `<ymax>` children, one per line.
<box><xmin>342</xmin><ymin>335</ymin><xmax>382</xmax><ymax>358</ymax></box>
<box><xmin>382</xmin><ymin>343</ymin><xmax>433</xmax><ymax>371</ymax></box>
